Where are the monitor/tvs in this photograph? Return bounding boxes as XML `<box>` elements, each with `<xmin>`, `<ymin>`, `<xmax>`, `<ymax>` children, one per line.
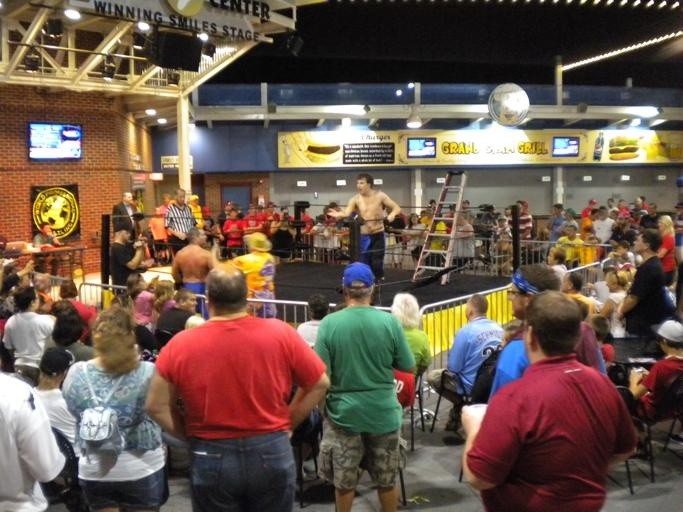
<box><xmin>407</xmin><ymin>137</ymin><xmax>436</xmax><ymax>158</ymax></box>
<box><xmin>27</xmin><ymin>121</ymin><xmax>83</xmax><ymax>161</ymax></box>
<box><xmin>552</xmin><ymin>136</ymin><xmax>579</xmax><ymax>156</ymax></box>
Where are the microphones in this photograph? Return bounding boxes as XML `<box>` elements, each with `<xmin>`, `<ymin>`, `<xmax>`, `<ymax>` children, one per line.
<box><xmin>127</xmin><ymin>239</ymin><xmax>147</xmax><ymax>246</ymax></box>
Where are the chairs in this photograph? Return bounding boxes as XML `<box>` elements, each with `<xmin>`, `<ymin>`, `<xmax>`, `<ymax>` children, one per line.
<box><xmin>155</xmin><ymin>329</ymin><xmax>172</xmax><ymax>350</ymax></box>
<box><xmin>612</xmin><ymin>386</ymin><xmax>635</xmax><ymax>495</ymax></box>
<box><xmin>291</xmin><ymin>345</ymin><xmax>504</xmax><ymax>508</ymax></box>
<box><xmin>644</xmin><ymin>370</ymin><xmax>683</xmax><ymax>483</ymax></box>
<box><xmin>41</xmin><ymin>427</ymin><xmax>85</xmax><ymax>512</ymax></box>
<box><xmin>136</xmin><ymin>325</ymin><xmax>154</xmax><ymax>349</ymax></box>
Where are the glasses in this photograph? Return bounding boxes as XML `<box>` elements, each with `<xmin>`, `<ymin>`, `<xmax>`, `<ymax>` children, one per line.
<box><xmin>507</xmin><ymin>287</ymin><xmax>524</xmax><ymax>300</ymax></box>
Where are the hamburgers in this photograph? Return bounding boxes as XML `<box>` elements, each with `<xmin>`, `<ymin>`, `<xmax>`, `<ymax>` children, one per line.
<box><xmin>609</xmin><ymin>137</ymin><xmax>640</xmax><ymax>160</ymax></box>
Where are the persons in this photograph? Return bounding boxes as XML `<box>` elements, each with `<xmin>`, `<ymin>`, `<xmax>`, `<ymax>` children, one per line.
<box><xmin>147</xmin><ymin>267</ymin><xmax>331</xmax><ymax>512</ymax></box>
<box><xmin>112</xmin><ymin>190</ymin><xmax>173</xmax><ymax>267</ymax></box>
<box><xmin>461</xmin><ymin>290</ymin><xmax>638</xmax><ymax>511</ymax></box>
<box><xmin>2</xmin><ymin>225</ymin><xmax>207</xmax><ymax>512</ymax></box>
<box><xmin>612</xmin><ymin>318</ymin><xmax>683</xmax><ymax>460</ymax></box>
<box><xmin>288</xmin><ymin>263</ymin><xmax>431</xmax><ymax>511</ymax></box>
<box><xmin>486</xmin><ymin>264</ymin><xmax>609</xmax><ymax>405</ymax></box>
<box><xmin>544</xmin><ymin>197</ymin><xmax>682</xmax><ymax>372</ymax></box>
<box><xmin>199</xmin><ymin>200</ymin><xmax>352</xmax><ymax>266</ymax></box>
<box><xmin>384</xmin><ymin>198</ymin><xmax>534</xmax><ymax>274</ymax></box>
<box><xmin>170</xmin><ymin>227</ymin><xmax>219</xmax><ymax>318</ymax></box>
<box><xmin>426</xmin><ymin>292</ymin><xmax>504</xmax><ymax>444</ymax></box>
<box><xmin>164</xmin><ymin>188</ymin><xmax>199</xmax><ymax>257</ymax></box>
<box><xmin>222</xmin><ymin>232</ymin><xmax>278</xmax><ymax>319</ymax></box>
<box><xmin>327</xmin><ymin>173</ymin><xmax>402</xmax><ymax>293</ymax></box>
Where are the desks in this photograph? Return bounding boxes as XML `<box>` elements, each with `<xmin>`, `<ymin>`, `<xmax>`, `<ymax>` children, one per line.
<box><xmin>601</xmin><ymin>337</ymin><xmax>665</xmax><ymax>387</ymax></box>
<box><xmin>29</xmin><ymin>246</ymin><xmax>86</xmax><ymax>284</ymax></box>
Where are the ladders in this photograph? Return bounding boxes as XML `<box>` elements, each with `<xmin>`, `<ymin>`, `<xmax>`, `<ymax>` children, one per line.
<box><xmin>413</xmin><ymin>170</ymin><xmax>467</xmax><ymax>286</ymax></box>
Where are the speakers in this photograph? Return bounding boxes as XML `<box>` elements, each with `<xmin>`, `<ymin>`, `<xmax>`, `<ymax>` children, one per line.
<box><xmin>145</xmin><ymin>30</ymin><xmax>202</xmax><ymax>72</ymax></box>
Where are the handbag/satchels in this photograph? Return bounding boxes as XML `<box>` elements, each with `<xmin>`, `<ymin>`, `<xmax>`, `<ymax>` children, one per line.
<box><xmin>393</xmin><ymin>367</ymin><xmax>415</xmax><ymax>408</ymax></box>
<box><xmin>630</xmin><ymin>366</ymin><xmax>650</xmax><ymax>400</ymax></box>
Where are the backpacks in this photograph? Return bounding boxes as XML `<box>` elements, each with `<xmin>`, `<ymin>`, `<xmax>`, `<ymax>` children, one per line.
<box><xmin>79</xmin><ymin>361</ymin><xmax>127</xmax><ymax>459</ymax></box>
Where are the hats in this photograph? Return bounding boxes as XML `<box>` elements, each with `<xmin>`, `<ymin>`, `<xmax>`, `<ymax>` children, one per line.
<box><xmin>343</xmin><ymin>262</ymin><xmax>376</xmax><ymax>289</ymax></box>
<box><xmin>650</xmin><ymin>320</ymin><xmax>683</xmax><ymax>343</ymax></box>
<box><xmin>190</xmin><ymin>195</ymin><xmax>199</xmax><ymax>200</ymax></box>
<box><xmin>564</xmin><ymin>207</ymin><xmax>577</xmax><ymax>217</ymax></box>
<box><xmin>114</xmin><ymin>222</ymin><xmax>134</xmax><ymax>231</ymax></box>
<box><xmin>589</xmin><ymin>199</ymin><xmax>598</xmax><ymax>204</ymax></box>
<box><xmin>243</xmin><ymin>232</ymin><xmax>272</xmax><ymax>252</ymax></box>
<box><xmin>498</xmin><ymin>215</ymin><xmax>507</xmax><ymax>221</ymax></box>
<box><xmin>41</xmin><ymin>347</ymin><xmax>76</xmax><ymax>376</ymax></box>
<box><xmin>307</xmin><ymin>293</ymin><xmax>328</xmax><ymax>311</ymax></box>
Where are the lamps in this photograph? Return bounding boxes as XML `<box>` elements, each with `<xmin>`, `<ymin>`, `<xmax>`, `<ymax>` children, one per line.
<box><xmin>407</xmin><ymin>107</ymin><xmax>422</xmax><ymax>128</ymax></box>
